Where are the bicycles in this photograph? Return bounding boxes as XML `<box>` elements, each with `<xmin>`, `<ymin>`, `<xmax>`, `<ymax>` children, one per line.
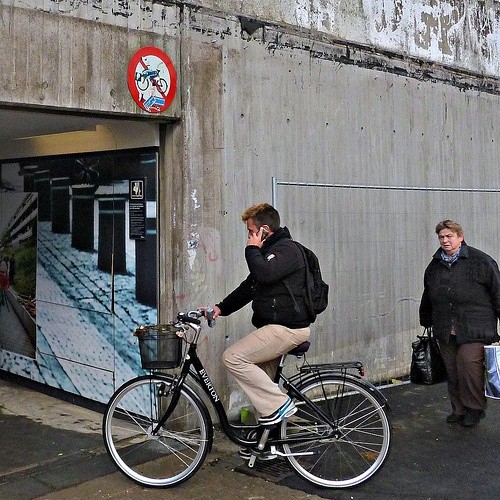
<box><xmin>100</xmin><ymin>308</ymin><xmax>395</xmax><ymax>489</ymax></box>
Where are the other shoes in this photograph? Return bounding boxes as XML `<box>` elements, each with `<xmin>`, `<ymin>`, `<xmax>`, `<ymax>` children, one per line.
<box><xmin>258</xmin><ymin>396</ymin><xmax>297</xmax><ymax>426</ymax></box>
<box><xmin>447</xmin><ymin>413</ymin><xmax>462</xmax><ymax>423</ymax></box>
<box><xmin>237</xmin><ymin>446</ymin><xmax>279</xmax><ymax>460</ymax></box>
<box><xmin>461</xmin><ymin>409</ymin><xmax>487</xmax><ymax>427</ymax></box>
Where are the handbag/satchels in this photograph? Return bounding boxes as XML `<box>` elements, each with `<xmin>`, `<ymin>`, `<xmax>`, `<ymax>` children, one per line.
<box><xmin>409</xmin><ymin>327</ymin><xmax>449</xmax><ymax>385</ymax></box>
<box><xmin>483</xmin><ymin>340</ymin><xmax>500</xmax><ymax>400</ymax></box>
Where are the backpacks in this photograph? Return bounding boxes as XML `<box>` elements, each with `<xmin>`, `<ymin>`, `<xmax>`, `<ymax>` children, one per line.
<box><xmin>267</xmin><ymin>241</ymin><xmax>329</xmax><ymax>323</ymax></box>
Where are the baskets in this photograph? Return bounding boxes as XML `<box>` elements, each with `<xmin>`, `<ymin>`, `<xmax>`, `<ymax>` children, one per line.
<box><xmin>134</xmin><ymin>324</ymin><xmax>182</xmax><ymax>370</ymax></box>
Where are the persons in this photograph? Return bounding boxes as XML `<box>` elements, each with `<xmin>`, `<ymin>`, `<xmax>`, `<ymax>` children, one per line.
<box><xmin>203</xmin><ymin>202</ymin><xmax>330</xmax><ymax>462</ymax></box>
<box><xmin>418</xmin><ymin>219</ymin><xmax>500</xmax><ymax>429</ymax></box>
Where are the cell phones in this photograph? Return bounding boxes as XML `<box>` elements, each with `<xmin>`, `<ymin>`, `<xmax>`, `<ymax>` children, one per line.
<box><xmin>256</xmin><ymin>228</ymin><xmax>268</xmax><ymax>241</ymax></box>
